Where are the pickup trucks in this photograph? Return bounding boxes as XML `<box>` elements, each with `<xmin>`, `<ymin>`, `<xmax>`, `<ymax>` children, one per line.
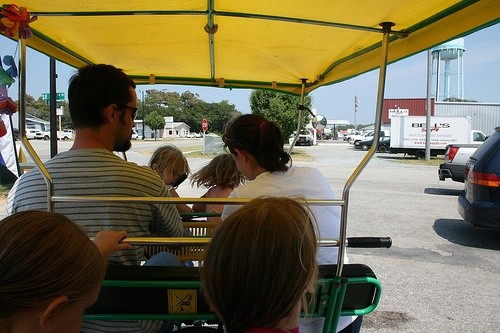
<box><xmin>438</xmin><ymin>127</ymin><xmax>500</xmax><ymax>230</ymax></box>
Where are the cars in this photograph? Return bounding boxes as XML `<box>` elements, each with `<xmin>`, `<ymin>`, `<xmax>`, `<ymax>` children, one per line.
<box><xmin>318</xmin><ymin>133</ymin><xmax>332</xmax><ymax>141</ymax></box>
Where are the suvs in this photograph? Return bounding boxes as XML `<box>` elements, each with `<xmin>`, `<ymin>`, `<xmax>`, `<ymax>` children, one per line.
<box><xmin>131</xmin><ymin>129</ymin><xmax>138</xmax><ymax>140</ymax></box>
<box><xmin>26</xmin><ymin>128</ymin><xmax>76</xmax><ymax>140</ymax></box>
<box><xmin>344</xmin><ymin>130</ymin><xmax>391</xmax><ymax>153</ymax></box>
<box><xmin>293</xmin><ymin>130</ymin><xmax>316</xmax><ymax>147</ymax></box>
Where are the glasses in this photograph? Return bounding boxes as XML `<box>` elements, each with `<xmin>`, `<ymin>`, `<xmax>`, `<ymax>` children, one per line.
<box><xmin>124</xmin><ymin>105</ymin><xmax>139</xmax><ymax>121</ymax></box>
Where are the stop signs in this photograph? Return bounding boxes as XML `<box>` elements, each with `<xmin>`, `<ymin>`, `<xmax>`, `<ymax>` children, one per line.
<box><xmin>202</xmin><ymin>118</ymin><xmax>208</xmax><ymax>131</ymax></box>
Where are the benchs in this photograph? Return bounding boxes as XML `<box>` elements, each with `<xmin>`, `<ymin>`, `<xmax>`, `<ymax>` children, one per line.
<box><xmin>54</xmin><ymin>199</ymin><xmax>381</xmax><ymax>333</ymax></box>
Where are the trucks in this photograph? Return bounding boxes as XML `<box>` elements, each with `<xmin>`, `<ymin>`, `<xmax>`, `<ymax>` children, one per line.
<box><xmin>389</xmin><ymin>115</ymin><xmax>489</xmax><ymax>159</ymax></box>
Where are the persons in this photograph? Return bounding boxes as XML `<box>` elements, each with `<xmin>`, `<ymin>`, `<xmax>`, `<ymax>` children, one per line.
<box><xmin>191</xmin><ymin>153</ymin><xmax>247</xmax><ymax>236</ymax></box>
<box><xmin>200</xmin><ymin>195</ymin><xmax>321</xmax><ymax>333</ymax></box>
<box><xmin>0</xmin><ymin>209</ymin><xmax>135</xmax><ymax>333</ymax></box>
<box><xmin>5</xmin><ymin>63</ymin><xmax>184</xmax><ymax>266</ymax></box>
<box><xmin>147</xmin><ymin>144</ymin><xmax>193</xmax><ymax>235</ymax></box>
<box><xmin>223</xmin><ymin>113</ymin><xmax>350</xmax><ymax>266</ymax></box>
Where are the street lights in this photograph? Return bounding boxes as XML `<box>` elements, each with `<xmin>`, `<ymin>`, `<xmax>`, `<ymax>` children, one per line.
<box><xmin>354</xmin><ymin>96</ymin><xmax>358</xmax><ymax>129</ymax></box>
